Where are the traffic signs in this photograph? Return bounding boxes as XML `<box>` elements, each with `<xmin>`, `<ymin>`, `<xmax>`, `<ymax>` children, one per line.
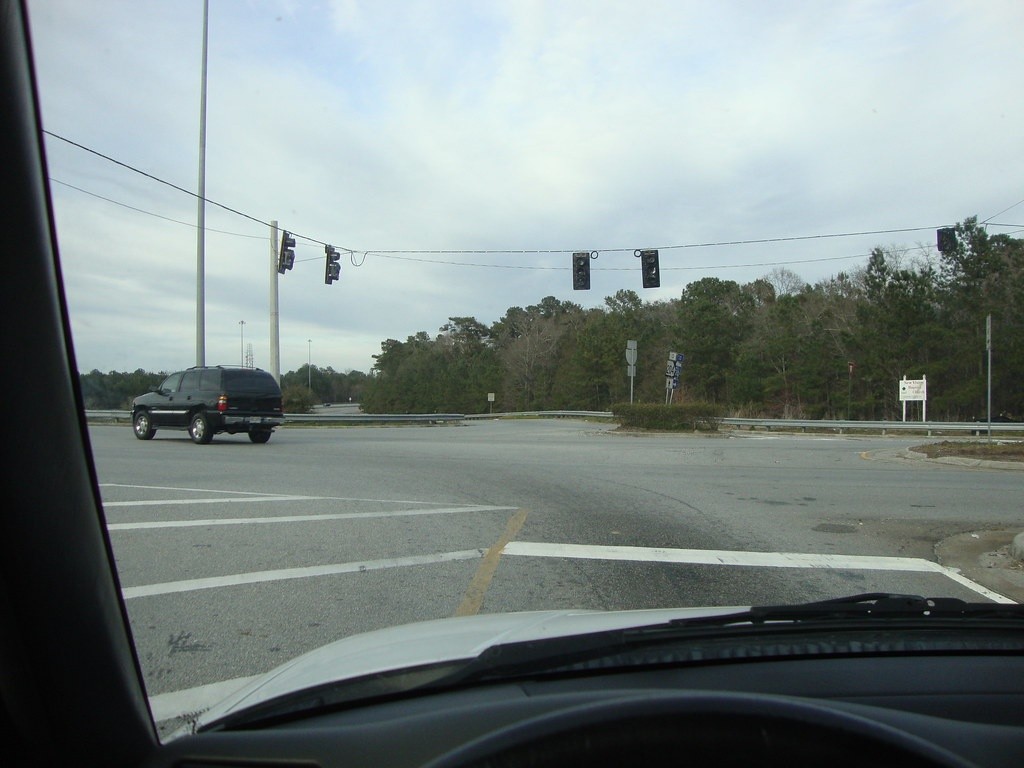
<box><xmin>987</xmin><ymin>315</ymin><xmax>991</xmax><ymax>349</ymax></box>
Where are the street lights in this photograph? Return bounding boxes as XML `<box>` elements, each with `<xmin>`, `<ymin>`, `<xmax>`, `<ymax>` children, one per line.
<box><xmin>239</xmin><ymin>321</ymin><xmax>247</xmax><ymax>368</ymax></box>
<box><xmin>307</xmin><ymin>339</ymin><xmax>313</xmax><ymax>392</ymax></box>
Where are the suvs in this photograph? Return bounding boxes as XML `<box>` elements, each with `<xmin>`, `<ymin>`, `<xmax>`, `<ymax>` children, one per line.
<box><xmin>132</xmin><ymin>365</ymin><xmax>284</xmax><ymax>445</ymax></box>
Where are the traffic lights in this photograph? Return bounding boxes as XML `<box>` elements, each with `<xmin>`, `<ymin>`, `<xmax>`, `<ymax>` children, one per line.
<box><xmin>279</xmin><ymin>230</ymin><xmax>295</xmax><ymax>273</ymax></box>
<box><xmin>937</xmin><ymin>229</ymin><xmax>956</xmax><ymax>252</ymax></box>
<box><xmin>642</xmin><ymin>250</ymin><xmax>660</xmax><ymax>289</ymax></box>
<box><xmin>325</xmin><ymin>245</ymin><xmax>340</xmax><ymax>285</ymax></box>
<box><xmin>573</xmin><ymin>252</ymin><xmax>591</xmax><ymax>290</ymax></box>
